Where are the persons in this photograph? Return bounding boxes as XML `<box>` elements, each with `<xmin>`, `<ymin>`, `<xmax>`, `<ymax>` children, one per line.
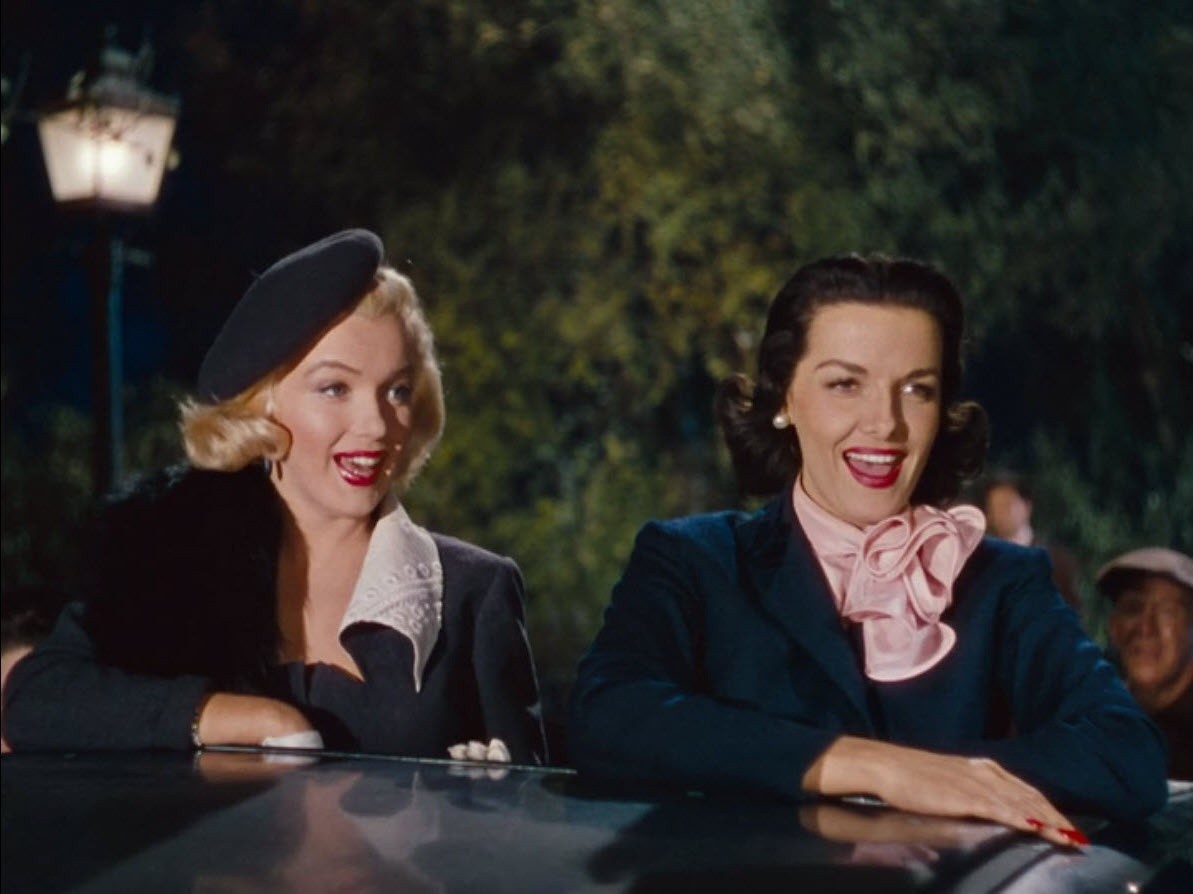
<box><xmin>1095</xmin><ymin>545</ymin><xmax>1193</xmax><ymax>782</ymax></box>
<box><xmin>554</xmin><ymin>253</ymin><xmax>1168</xmax><ymax>845</ymax></box>
<box><xmin>1</xmin><ymin>229</ymin><xmax>550</xmax><ymax>765</ymax></box>
<box><xmin>982</xmin><ymin>478</ymin><xmax>1081</xmax><ymax>601</ymax></box>
<box><xmin>1</xmin><ymin>752</ymin><xmax>1012</xmax><ymax>894</ymax></box>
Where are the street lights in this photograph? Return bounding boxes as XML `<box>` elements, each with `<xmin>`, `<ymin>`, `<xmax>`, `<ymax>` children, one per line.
<box><xmin>36</xmin><ymin>40</ymin><xmax>183</xmax><ymax>487</ymax></box>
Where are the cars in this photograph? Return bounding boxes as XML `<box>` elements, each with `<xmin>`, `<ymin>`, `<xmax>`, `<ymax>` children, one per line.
<box><xmin>0</xmin><ymin>738</ymin><xmax>1180</xmax><ymax>894</ymax></box>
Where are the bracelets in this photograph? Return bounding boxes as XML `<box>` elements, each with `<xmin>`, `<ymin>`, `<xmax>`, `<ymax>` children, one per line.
<box><xmin>190</xmin><ymin>693</ymin><xmax>207</xmax><ymax>747</ymax></box>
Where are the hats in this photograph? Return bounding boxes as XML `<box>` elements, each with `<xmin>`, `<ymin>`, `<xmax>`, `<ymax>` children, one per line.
<box><xmin>1093</xmin><ymin>547</ymin><xmax>1193</xmax><ymax>592</ymax></box>
<box><xmin>198</xmin><ymin>227</ymin><xmax>381</xmax><ymax>405</ymax></box>
